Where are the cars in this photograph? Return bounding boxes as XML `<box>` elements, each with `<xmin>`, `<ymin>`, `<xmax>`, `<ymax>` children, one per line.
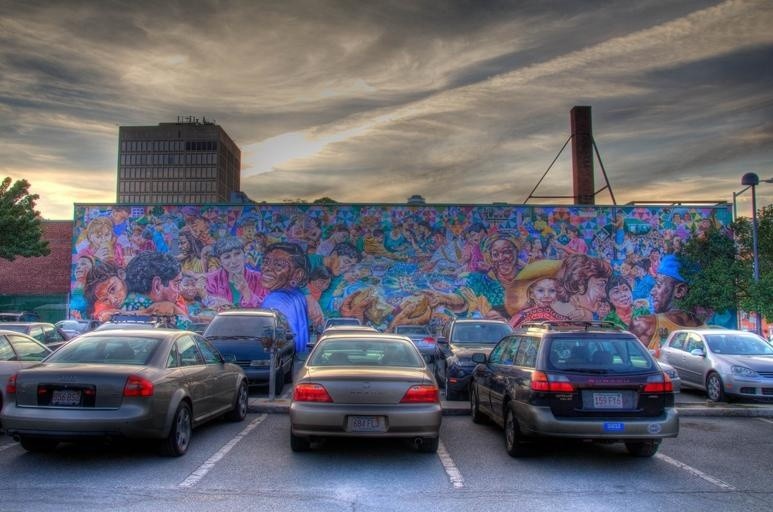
<box><xmin>283</xmin><ymin>334</ymin><xmax>443</xmax><ymax>455</ymax></box>
<box><xmin>2</xmin><ymin>312</ymin><xmax>180</xmax><ymax>351</ymax></box>
<box><xmin>320</xmin><ymin>316</ymin><xmax>379</xmax><ymax>336</ymax></box>
<box><xmin>391</xmin><ymin>324</ymin><xmax>439</xmax><ymax>358</ymax></box>
<box><xmin>658</xmin><ymin>329</ymin><xmax>771</xmax><ymax>402</ymax></box>
<box><xmin>1</xmin><ymin>329</ymin><xmax>251</xmax><ymax>456</ymax></box>
<box><xmin>1</xmin><ymin>328</ymin><xmax>56</xmax><ymax>406</ymax></box>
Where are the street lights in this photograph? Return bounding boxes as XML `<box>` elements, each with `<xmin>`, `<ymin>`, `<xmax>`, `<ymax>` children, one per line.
<box><xmin>731</xmin><ymin>177</ymin><xmax>772</xmax><ymax>221</ymax></box>
<box><xmin>740</xmin><ymin>173</ymin><xmax>761</xmax><ymax>337</ymax></box>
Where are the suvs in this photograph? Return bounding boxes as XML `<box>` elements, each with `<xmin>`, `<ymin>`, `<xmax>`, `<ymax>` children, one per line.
<box><xmin>203</xmin><ymin>310</ymin><xmax>297</xmax><ymax>395</ymax></box>
<box><xmin>433</xmin><ymin>315</ymin><xmax>515</xmax><ymax>399</ymax></box>
<box><xmin>467</xmin><ymin>316</ymin><xmax>682</xmax><ymax>459</ymax></box>
